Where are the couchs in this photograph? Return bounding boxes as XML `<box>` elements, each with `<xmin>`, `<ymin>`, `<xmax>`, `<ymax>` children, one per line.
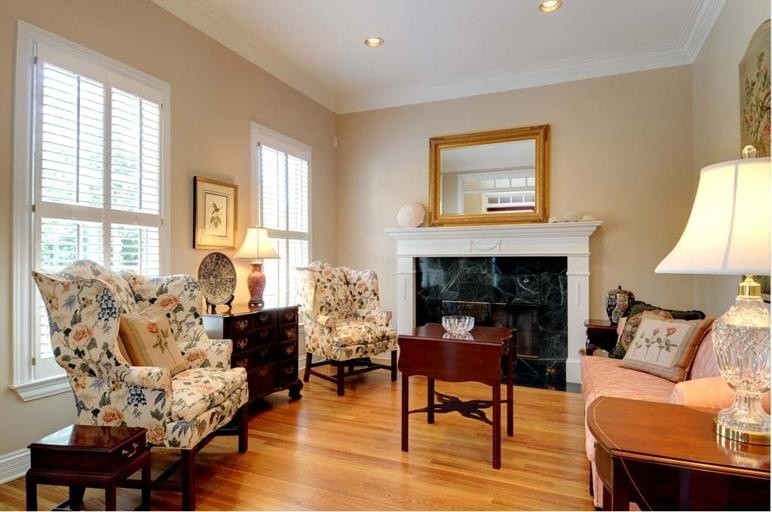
<box><xmin>580</xmin><ymin>326</ymin><xmax>770</xmax><ymax>511</ymax></box>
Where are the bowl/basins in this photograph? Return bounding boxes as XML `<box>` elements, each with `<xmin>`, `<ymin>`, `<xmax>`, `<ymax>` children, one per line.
<box><xmin>442</xmin><ymin>330</ymin><xmax>475</xmax><ymax>342</ymax></box>
<box><xmin>441</xmin><ymin>314</ymin><xmax>476</xmax><ymax>333</ymax></box>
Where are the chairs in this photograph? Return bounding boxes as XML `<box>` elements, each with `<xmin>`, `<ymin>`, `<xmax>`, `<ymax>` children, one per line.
<box><xmin>295</xmin><ymin>260</ymin><xmax>399</xmax><ymax>396</ymax></box>
<box><xmin>31</xmin><ymin>260</ymin><xmax>250</xmax><ymax>511</ymax></box>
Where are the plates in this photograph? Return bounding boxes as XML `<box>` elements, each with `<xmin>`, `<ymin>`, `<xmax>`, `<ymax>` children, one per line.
<box><xmin>197</xmin><ymin>250</ymin><xmax>238</xmax><ymax>306</ymax></box>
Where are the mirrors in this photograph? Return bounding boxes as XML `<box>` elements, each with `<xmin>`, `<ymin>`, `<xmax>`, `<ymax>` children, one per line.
<box><xmin>426</xmin><ymin>124</ymin><xmax>549</xmax><ymax>227</ymax></box>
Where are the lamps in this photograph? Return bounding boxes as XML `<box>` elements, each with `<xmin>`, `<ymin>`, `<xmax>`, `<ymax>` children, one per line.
<box><xmin>539</xmin><ymin>0</ymin><xmax>562</xmax><ymax>13</ymax></box>
<box><xmin>365</xmin><ymin>38</ymin><xmax>383</xmax><ymax>49</ymax></box>
<box><xmin>654</xmin><ymin>144</ymin><xmax>771</xmax><ymax>454</ymax></box>
<box><xmin>233</xmin><ymin>227</ymin><xmax>280</xmax><ymax>311</ymax></box>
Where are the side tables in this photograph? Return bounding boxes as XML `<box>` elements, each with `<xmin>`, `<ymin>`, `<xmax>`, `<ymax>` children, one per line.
<box><xmin>587</xmin><ymin>395</ymin><xmax>771</xmax><ymax>511</ymax></box>
<box><xmin>583</xmin><ymin>318</ymin><xmax>618</xmax><ymax>357</ymax></box>
<box><xmin>26</xmin><ymin>425</ymin><xmax>150</xmax><ymax>511</ymax></box>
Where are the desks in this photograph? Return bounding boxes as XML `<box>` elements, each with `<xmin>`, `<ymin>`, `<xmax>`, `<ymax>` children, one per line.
<box><xmin>398</xmin><ymin>323</ymin><xmax>517</xmax><ymax>469</ymax></box>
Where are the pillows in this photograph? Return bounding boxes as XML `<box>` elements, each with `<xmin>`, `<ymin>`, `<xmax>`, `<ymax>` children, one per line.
<box><xmin>608</xmin><ymin>301</ymin><xmax>716</xmax><ymax>383</ymax></box>
<box><xmin>118</xmin><ymin>299</ymin><xmax>189</xmax><ymax>378</ymax></box>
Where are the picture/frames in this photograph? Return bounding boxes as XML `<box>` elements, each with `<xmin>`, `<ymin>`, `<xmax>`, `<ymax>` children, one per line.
<box><xmin>192</xmin><ymin>176</ymin><xmax>238</xmax><ymax>253</ymax></box>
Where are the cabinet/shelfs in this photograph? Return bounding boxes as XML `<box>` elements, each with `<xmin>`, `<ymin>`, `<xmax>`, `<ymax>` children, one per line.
<box><xmin>198</xmin><ymin>303</ymin><xmax>304</xmax><ymax>415</ymax></box>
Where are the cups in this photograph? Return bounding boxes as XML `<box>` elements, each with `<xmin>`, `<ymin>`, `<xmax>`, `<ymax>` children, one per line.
<box><xmin>548</xmin><ymin>211</ymin><xmax>596</xmax><ymax>224</ymax></box>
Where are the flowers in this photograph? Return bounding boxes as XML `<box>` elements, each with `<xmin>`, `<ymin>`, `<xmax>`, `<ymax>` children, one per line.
<box><xmin>63</xmin><ymin>323</ymin><xmax>109</xmax><ymax>380</ymax></box>
<box><xmin>155</xmin><ymin>293</ymin><xmax>190</xmax><ymax>341</ymax></box>
<box><xmin>146</xmin><ymin>320</ymin><xmax>179</xmax><ymax>367</ymax></box>
<box><xmin>96</xmin><ymin>405</ymin><xmax>127</xmax><ymax>427</ymax></box>
<box><xmin>183</xmin><ymin>349</ymin><xmax>209</xmax><ymax>368</ymax></box>
<box><xmin>143</xmin><ymin>417</ymin><xmax>168</xmax><ymax>450</ymax></box>
